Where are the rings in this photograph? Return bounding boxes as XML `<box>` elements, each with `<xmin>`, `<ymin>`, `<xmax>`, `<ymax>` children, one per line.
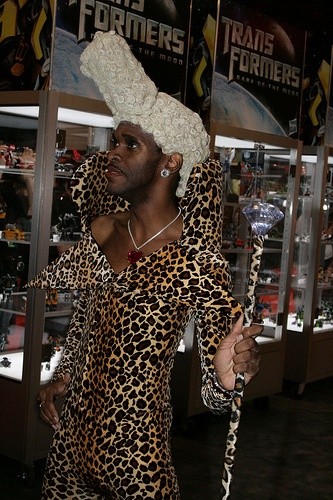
<box><xmin>38</xmin><ymin>398</ymin><xmax>46</xmax><ymax>407</ymax></box>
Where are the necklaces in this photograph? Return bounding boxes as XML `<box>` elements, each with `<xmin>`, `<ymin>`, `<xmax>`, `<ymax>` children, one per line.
<box><xmin>126</xmin><ymin>207</ymin><xmax>182</xmax><ymax>262</ymax></box>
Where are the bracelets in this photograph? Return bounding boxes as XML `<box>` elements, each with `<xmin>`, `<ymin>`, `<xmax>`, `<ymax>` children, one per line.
<box><xmin>212</xmin><ymin>371</ymin><xmax>235</xmax><ymax>397</ymax></box>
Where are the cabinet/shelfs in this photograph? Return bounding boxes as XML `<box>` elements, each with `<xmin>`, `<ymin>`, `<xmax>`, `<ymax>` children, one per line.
<box><xmin>0</xmin><ymin>89</ymin><xmax>333</xmax><ymax>474</ymax></box>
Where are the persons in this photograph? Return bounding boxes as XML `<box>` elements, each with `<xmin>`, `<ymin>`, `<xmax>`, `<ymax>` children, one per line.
<box><xmin>0</xmin><ymin>143</ymin><xmax>34</xmax><ymax>352</ymax></box>
<box><xmin>37</xmin><ymin>89</ymin><xmax>266</xmax><ymax>500</ymax></box>
